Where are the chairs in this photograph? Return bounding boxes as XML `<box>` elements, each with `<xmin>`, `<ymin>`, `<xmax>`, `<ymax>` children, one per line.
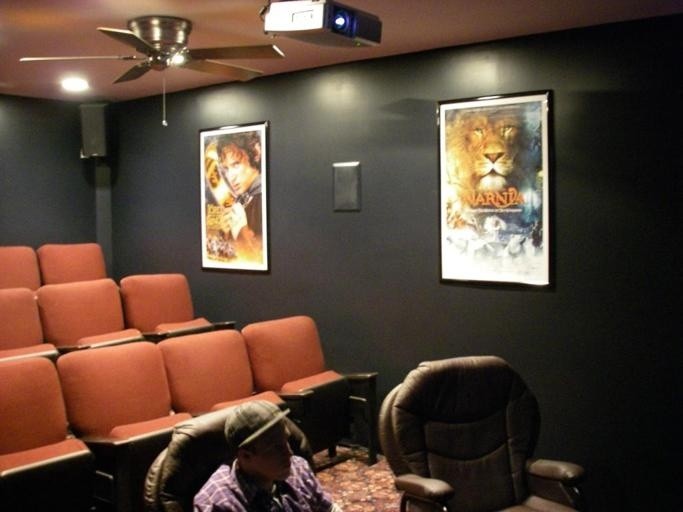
<box><xmin>0</xmin><ymin>241</ymin><xmax>212</xmax><ymax>512</ymax></box>
<box><xmin>378</xmin><ymin>355</ymin><xmax>586</xmax><ymax>512</ymax></box>
<box><xmin>154</xmin><ymin>330</ymin><xmax>287</xmax><ymax>432</ymax></box>
<box><xmin>144</xmin><ymin>404</ymin><xmax>315</xmax><ymax>512</ymax></box>
<box><xmin>243</xmin><ymin>316</ymin><xmax>380</xmax><ymax>473</ymax></box>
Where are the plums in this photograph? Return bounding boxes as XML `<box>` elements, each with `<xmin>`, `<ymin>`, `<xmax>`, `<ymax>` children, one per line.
<box><xmin>264</xmin><ymin>0</ymin><xmax>382</xmax><ymax>48</ymax></box>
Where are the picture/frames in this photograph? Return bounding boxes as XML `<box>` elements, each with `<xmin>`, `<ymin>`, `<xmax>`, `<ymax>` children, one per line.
<box><xmin>198</xmin><ymin>120</ymin><xmax>271</xmax><ymax>276</ymax></box>
<box><xmin>436</xmin><ymin>88</ymin><xmax>556</xmax><ymax>292</ymax></box>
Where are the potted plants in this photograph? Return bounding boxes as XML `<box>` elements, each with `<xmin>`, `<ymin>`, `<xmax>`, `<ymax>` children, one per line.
<box><xmin>436</xmin><ymin>88</ymin><xmax>556</xmax><ymax>292</ymax></box>
<box><xmin>198</xmin><ymin>120</ymin><xmax>271</xmax><ymax>276</ymax></box>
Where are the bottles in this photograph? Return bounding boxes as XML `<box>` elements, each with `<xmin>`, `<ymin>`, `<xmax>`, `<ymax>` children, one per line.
<box><xmin>224</xmin><ymin>399</ymin><xmax>291</xmax><ymax>453</ymax></box>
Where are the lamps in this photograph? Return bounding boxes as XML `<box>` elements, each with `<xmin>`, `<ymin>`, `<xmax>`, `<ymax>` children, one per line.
<box><xmin>378</xmin><ymin>355</ymin><xmax>586</xmax><ymax>512</ymax></box>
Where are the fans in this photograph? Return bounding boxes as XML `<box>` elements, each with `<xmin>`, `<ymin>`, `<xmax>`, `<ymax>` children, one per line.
<box><xmin>17</xmin><ymin>15</ymin><xmax>285</xmax><ymax>128</ymax></box>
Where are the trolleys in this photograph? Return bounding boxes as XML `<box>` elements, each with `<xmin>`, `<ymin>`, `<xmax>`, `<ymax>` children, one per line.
<box><xmin>17</xmin><ymin>15</ymin><xmax>285</xmax><ymax>128</ymax></box>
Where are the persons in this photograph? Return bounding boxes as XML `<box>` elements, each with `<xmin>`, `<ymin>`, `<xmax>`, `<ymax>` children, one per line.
<box><xmin>193</xmin><ymin>400</ymin><xmax>335</xmax><ymax>512</ymax></box>
<box><xmin>216</xmin><ymin>133</ymin><xmax>262</xmax><ymax>258</ymax></box>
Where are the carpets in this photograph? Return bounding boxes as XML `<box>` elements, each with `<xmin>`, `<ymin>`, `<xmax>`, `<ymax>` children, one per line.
<box><xmin>307</xmin><ymin>446</ymin><xmax>401</xmax><ymax>512</ymax></box>
